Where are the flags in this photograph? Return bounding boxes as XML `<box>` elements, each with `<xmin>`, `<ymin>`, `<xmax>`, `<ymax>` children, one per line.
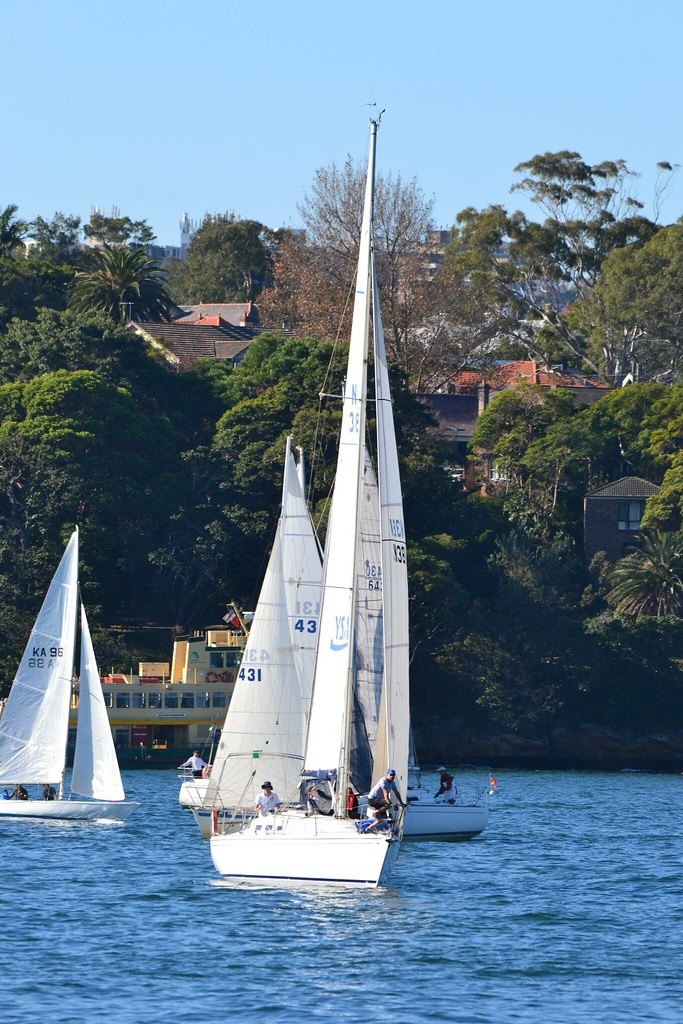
<box><xmin>223</xmin><ymin>609</ymin><xmax>240</xmax><ymax>629</ymax></box>
<box><xmin>490</xmin><ymin>775</ymin><xmax>496</xmax><ymax>786</ymax></box>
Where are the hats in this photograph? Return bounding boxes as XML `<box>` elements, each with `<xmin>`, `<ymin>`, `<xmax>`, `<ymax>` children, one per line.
<box><xmin>387</xmin><ymin>769</ymin><xmax>395</xmax><ymax>775</ymax></box>
<box><xmin>306</xmin><ymin>785</ymin><xmax>316</xmax><ymax>792</ymax></box>
<box><xmin>437</xmin><ymin>767</ymin><xmax>446</xmax><ymax>772</ymax></box>
<box><xmin>262</xmin><ymin>781</ymin><xmax>273</xmax><ymax>791</ymax></box>
<box><xmin>192</xmin><ymin>748</ymin><xmax>201</xmax><ymax>752</ymax></box>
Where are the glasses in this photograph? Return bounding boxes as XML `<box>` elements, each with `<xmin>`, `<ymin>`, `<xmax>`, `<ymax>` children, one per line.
<box><xmin>389</xmin><ymin>774</ymin><xmax>395</xmax><ymax>776</ymax></box>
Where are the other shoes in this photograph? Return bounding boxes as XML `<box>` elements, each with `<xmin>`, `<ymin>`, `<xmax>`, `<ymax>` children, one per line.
<box><xmin>373</xmin><ymin>813</ymin><xmax>383</xmax><ymax>821</ymax></box>
<box><xmin>366</xmin><ymin>827</ymin><xmax>377</xmax><ymax>834</ymax></box>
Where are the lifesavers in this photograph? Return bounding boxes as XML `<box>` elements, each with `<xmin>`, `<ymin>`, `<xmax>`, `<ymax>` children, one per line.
<box><xmin>220</xmin><ymin>671</ymin><xmax>232</xmax><ymax>682</ymax></box>
<box><xmin>206</xmin><ymin>672</ymin><xmax>219</xmax><ymax>682</ymax></box>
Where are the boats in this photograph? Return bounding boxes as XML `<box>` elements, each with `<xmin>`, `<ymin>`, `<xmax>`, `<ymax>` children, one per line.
<box><xmin>67</xmin><ymin>600</ymin><xmax>253</xmax><ymax>765</ymax></box>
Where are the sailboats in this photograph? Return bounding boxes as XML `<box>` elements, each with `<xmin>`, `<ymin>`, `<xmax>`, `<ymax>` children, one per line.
<box><xmin>179</xmin><ymin>125</ymin><xmax>490</xmax><ymax>890</ymax></box>
<box><xmin>1</xmin><ymin>524</ymin><xmax>142</xmax><ymax>825</ymax></box>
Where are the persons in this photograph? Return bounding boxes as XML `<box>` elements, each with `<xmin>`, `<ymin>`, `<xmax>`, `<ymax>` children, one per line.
<box><xmin>0</xmin><ymin>789</ymin><xmax>9</xmax><ymax>800</ymax></box>
<box><xmin>180</xmin><ymin>750</ymin><xmax>208</xmax><ymax>776</ymax></box>
<box><xmin>432</xmin><ymin>767</ymin><xmax>455</xmax><ymax>798</ymax></box>
<box><xmin>42</xmin><ymin>784</ymin><xmax>56</xmax><ymax>800</ymax></box>
<box><xmin>305</xmin><ymin>785</ymin><xmax>335</xmax><ymax>818</ymax></box>
<box><xmin>9</xmin><ymin>785</ymin><xmax>28</xmax><ymax>800</ymax></box>
<box><xmin>140</xmin><ymin>741</ymin><xmax>143</xmax><ymax>748</ymax></box>
<box><xmin>342</xmin><ymin>787</ymin><xmax>359</xmax><ymax>820</ymax></box>
<box><xmin>2</xmin><ymin>697</ymin><xmax>7</xmax><ymax>706</ymax></box>
<box><xmin>254</xmin><ymin>781</ymin><xmax>281</xmax><ymax>817</ymax></box>
<box><xmin>364</xmin><ymin>769</ymin><xmax>403</xmax><ymax>834</ymax></box>
